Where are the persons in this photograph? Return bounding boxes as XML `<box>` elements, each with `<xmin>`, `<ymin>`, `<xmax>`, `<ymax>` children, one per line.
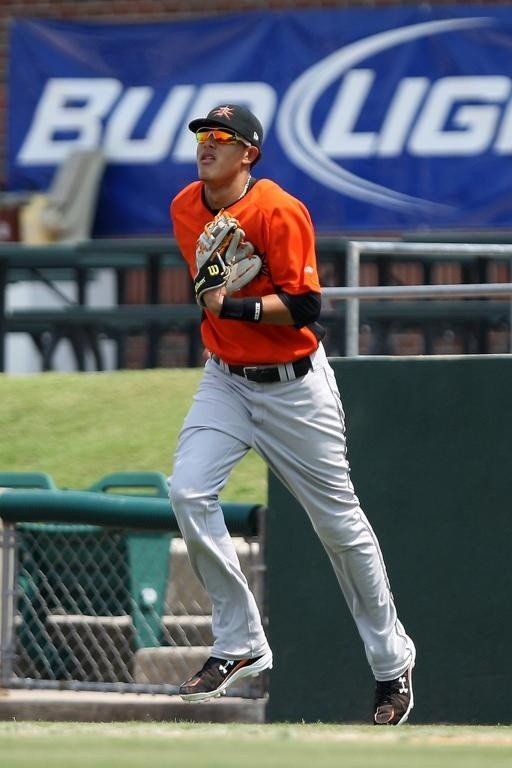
<box><xmin>165</xmin><ymin>100</ymin><xmax>418</xmax><ymax>727</ymax></box>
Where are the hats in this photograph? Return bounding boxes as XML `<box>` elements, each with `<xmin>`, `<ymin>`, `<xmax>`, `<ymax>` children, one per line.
<box><xmin>189</xmin><ymin>104</ymin><xmax>262</xmax><ymax>148</ymax></box>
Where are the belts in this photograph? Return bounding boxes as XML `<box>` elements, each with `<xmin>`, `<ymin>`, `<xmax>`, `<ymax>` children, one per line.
<box><xmin>210</xmin><ymin>353</ymin><xmax>310</xmax><ymax>383</ymax></box>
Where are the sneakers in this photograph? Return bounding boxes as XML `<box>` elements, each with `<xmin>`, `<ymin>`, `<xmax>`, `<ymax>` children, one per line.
<box><xmin>372</xmin><ymin>640</ymin><xmax>416</xmax><ymax>727</ymax></box>
<box><xmin>179</xmin><ymin>650</ymin><xmax>272</xmax><ymax>701</ymax></box>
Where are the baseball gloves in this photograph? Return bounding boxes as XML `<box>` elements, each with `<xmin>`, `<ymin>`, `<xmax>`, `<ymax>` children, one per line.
<box><xmin>193</xmin><ymin>208</ymin><xmax>263</xmax><ymax>306</ymax></box>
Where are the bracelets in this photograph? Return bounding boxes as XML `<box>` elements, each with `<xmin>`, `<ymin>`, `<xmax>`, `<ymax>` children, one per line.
<box><xmin>216</xmin><ymin>294</ymin><xmax>264</xmax><ymax>323</ymax></box>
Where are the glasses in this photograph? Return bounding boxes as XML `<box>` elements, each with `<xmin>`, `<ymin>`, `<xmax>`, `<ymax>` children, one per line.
<box><xmin>197</xmin><ymin>127</ymin><xmax>253</xmax><ymax>146</ymax></box>
